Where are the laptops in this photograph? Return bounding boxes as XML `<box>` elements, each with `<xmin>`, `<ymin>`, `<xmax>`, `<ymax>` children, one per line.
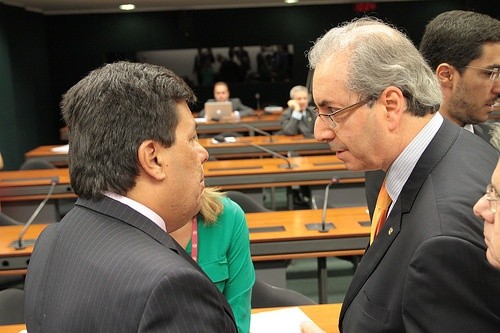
<box><xmin>204</xmin><ymin>102</ymin><xmax>234</xmax><ymax>121</ymax></box>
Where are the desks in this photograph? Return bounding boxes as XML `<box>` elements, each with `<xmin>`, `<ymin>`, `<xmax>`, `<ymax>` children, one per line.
<box><xmin>0</xmin><ymin>205</ymin><xmax>372</xmax><ymax>305</ymax></box>
<box><xmin>24</xmin><ymin>134</ymin><xmax>336</xmax><ymax>166</ymax></box>
<box><xmin>0</xmin><ymin>155</ymin><xmax>391</xmax><ymax>209</ymax></box>
<box><xmin>61</xmin><ymin>116</ymin><xmax>298</xmax><ymax>141</ymax></box>
<box><xmin>0</xmin><ymin>302</ymin><xmax>343</xmax><ymax>333</ymax></box>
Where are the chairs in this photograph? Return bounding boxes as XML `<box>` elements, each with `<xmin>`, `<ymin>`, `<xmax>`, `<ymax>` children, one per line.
<box><xmin>218</xmin><ymin>191</ymin><xmax>292</xmax><ymax>289</ymax></box>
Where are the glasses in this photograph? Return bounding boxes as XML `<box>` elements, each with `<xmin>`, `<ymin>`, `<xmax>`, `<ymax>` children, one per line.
<box><xmin>312</xmin><ymin>97</ymin><xmax>376</xmax><ymax>127</ymax></box>
<box><xmin>485</xmin><ymin>184</ymin><xmax>500</xmax><ymax>202</ymax></box>
<box><xmin>455</xmin><ymin>64</ymin><xmax>500</xmax><ymax>81</ymax></box>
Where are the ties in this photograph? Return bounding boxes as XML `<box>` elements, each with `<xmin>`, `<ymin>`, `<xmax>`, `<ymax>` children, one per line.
<box><xmin>369</xmin><ymin>177</ymin><xmax>393</xmax><ymax>246</ymax></box>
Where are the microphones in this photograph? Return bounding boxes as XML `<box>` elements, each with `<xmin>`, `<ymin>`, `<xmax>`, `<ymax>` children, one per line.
<box><xmin>214</xmin><ymin>134</ymin><xmax>291</xmax><ymax>169</ymax></box>
<box><xmin>15</xmin><ymin>176</ymin><xmax>59</xmax><ymax>250</ymax></box>
<box><xmin>210</xmin><ymin>117</ymin><xmax>272</xmax><ymax>142</ymax></box>
<box><xmin>255</xmin><ymin>92</ymin><xmax>262</xmax><ymax>119</ymax></box>
<box><xmin>318</xmin><ymin>176</ymin><xmax>340</xmax><ymax>233</ymax></box>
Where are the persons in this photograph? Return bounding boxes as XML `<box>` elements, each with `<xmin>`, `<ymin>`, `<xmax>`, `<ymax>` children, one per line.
<box><xmin>281</xmin><ymin>85</ymin><xmax>318</xmax><ymax>136</ymax></box>
<box><xmin>192</xmin><ymin>44</ymin><xmax>296</xmax><ymax>80</ymax></box>
<box><xmin>414</xmin><ymin>9</ymin><xmax>500</xmax><ymax>150</ymax></box>
<box><xmin>197</xmin><ymin>81</ymin><xmax>256</xmax><ymax>137</ymax></box>
<box><xmin>306</xmin><ymin>18</ymin><xmax>500</xmax><ymax>332</ymax></box>
<box><xmin>25</xmin><ymin>62</ymin><xmax>236</xmax><ymax>333</ymax></box>
<box><xmin>471</xmin><ymin>161</ymin><xmax>500</xmax><ymax>270</ymax></box>
<box><xmin>169</xmin><ymin>187</ymin><xmax>256</xmax><ymax>333</ymax></box>
